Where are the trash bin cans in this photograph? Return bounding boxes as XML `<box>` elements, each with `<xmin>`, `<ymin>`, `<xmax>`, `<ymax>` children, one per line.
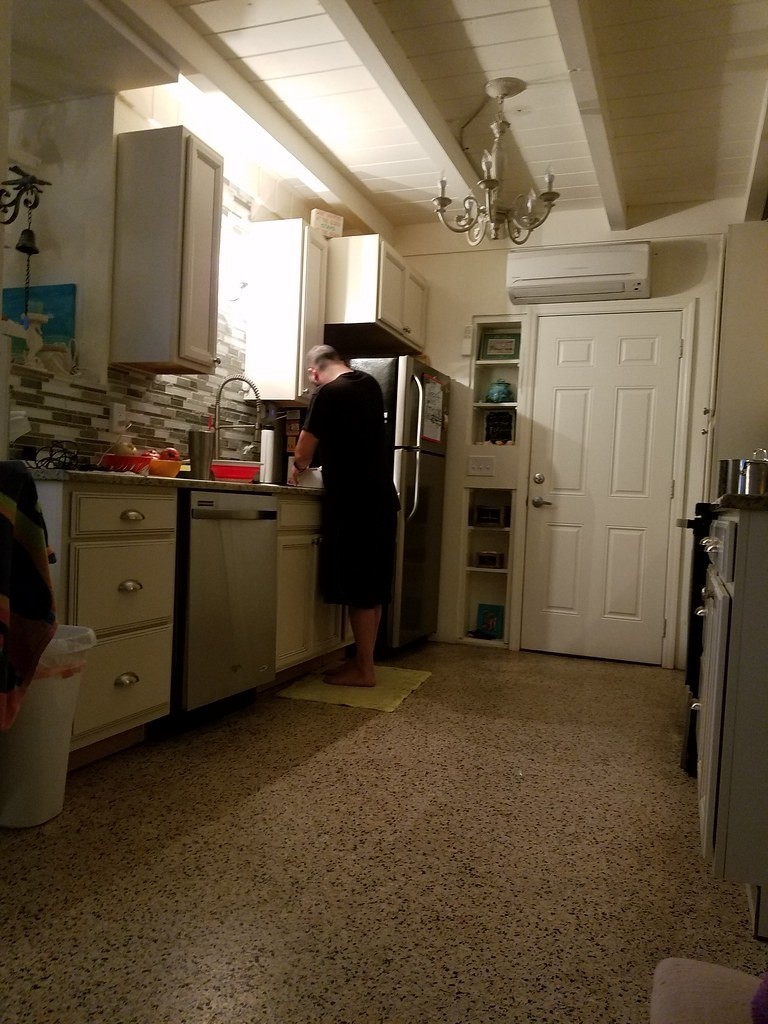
<box><xmin>0</xmin><ymin>624</ymin><xmax>95</xmax><ymax>830</ymax></box>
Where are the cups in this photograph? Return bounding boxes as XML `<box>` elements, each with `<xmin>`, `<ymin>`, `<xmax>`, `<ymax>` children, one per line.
<box><xmin>188</xmin><ymin>428</ymin><xmax>216</xmax><ymax>480</ymax></box>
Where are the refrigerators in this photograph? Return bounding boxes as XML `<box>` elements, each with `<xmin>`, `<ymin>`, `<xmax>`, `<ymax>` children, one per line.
<box><xmin>347</xmin><ymin>355</ymin><xmax>451</xmax><ymax>649</ymax></box>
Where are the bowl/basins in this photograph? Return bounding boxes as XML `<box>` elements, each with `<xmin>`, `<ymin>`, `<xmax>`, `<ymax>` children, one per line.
<box><xmin>149</xmin><ymin>459</ymin><xmax>183</xmax><ymax>478</ymax></box>
<box><xmin>101</xmin><ymin>453</ymin><xmax>154</xmax><ymax>474</ymax></box>
<box><xmin>210</xmin><ymin>459</ymin><xmax>264</xmax><ymax>483</ymax></box>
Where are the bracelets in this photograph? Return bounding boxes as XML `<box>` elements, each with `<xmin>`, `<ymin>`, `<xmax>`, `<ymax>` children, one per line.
<box><xmin>294</xmin><ymin>461</ymin><xmax>306</xmax><ymax>472</ymax></box>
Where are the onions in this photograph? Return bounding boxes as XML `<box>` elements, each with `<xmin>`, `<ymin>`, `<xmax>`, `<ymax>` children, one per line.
<box><xmin>116</xmin><ymin>442</ymin><xmax>138</xmax><ymax>456</ymax></box>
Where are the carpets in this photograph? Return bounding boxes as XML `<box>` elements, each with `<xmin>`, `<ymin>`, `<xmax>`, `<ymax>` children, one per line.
<box><xmin>275</xmin><ymin>662</ymin><xmax>432</xmax><ymax>713</ymax></box>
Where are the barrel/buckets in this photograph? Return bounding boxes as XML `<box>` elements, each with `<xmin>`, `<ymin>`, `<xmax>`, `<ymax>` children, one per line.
<box><xmin>717</xmin><ymin>448</ymin><xmax>768</xmax><ymax>499</ymax></box>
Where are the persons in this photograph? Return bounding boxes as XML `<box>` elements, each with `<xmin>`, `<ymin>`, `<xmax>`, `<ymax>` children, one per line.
<box><xmin>291</xmin><ymin>344</ymin><xmax>401</xmax><ymax>686</ymax></box>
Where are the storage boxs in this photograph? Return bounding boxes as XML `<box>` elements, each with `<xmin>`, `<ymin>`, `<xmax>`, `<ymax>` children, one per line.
<box><xmin>476</xmin><ymin>604</ymin><xmax>504</xmax><ymax>639</ymax></box>
<box><xmin>479</xmin><ymin>332</ymin><xmax>520</xmax><ymax>360</ymax></box>
<box><xmin>310</xmin><ymin>207</ymin><xmax>344</xmax><ymax>239</ymax></box>
<box><xmin>474</xmin><ymin>550</ymin><xmax>505</xmax><ymax>569</ymax></box>
<box><xmin>473</xmin><ymin>504</ymin><xmax>510</xmax><ymax>528</ymax></box>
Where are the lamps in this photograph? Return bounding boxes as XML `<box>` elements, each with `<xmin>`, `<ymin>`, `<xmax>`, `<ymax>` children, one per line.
<box><xmin>430</xmin><ymin>76</ymin><xmax>560</xmax><ymax>247</ymax></box>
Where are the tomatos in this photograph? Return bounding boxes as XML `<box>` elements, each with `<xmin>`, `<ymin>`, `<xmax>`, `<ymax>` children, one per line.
<box><xmin>141</xmin><ymin>447</ymin><xmax>180</xmax><ymax>460</ymax></box>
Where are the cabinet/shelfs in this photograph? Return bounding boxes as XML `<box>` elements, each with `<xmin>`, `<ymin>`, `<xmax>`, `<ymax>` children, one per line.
<box><xmin>109</xmin><ymin>124</ymin><xmax>225</xmax><ymax>377</ymax></box>
<box><xmin>237</xmin><ymin>218</ymin><xmax>330</xmax><ymax>409</ymax></box>
<box><xmin>688</xmin><ymin>508</ymin><xmax>768</xmax><ymax>942</ymax></box>
<box><xmin>465</xmin><ymin>315</ymin><xmax>527</xmax><ymax>450</ymax></box>
<box><xmin>61</xmin><ymin>480</ymin><xmax>177</xmax><ymax>775</ymax></box>
<box><xmin>325</xmin><ymin>233</ymin><xmax>432</xmax><ymax>355</ymax></box>
<box><xmin>277</xmin><ymin>494</ymin><xmax>357</xmax><ymax>683</ymax></box>
<box><xmin>458</xmin><ymin>483</ymin><xmax>518</xmax><ymax>650</ymax></box>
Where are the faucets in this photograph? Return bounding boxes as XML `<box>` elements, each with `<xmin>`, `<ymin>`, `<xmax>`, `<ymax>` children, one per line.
<box><xmin>213</xmin><ymin>374</ymin><xmax>262</xmax><ymax>461</ymax></box>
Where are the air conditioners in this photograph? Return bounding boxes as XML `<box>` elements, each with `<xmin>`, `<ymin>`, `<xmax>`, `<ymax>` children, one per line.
<box><xmin>504</xmin><ymin>238</ymin><xmax>651</xmax><ymax>306</ymax></box>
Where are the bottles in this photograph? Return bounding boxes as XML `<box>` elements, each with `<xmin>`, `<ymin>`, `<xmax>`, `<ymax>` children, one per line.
<box><xmin>259</xmin><ymin>421</ymin><xmax>274</xmax><ymax>484</ymax></box>
<box><xmin>486</xmin><ymin>378</ymin><xmax>512</xmax><ymax>403</ymax></box>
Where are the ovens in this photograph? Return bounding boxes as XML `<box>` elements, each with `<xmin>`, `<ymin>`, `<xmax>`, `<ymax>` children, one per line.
<box><xmin>691</xmin><ymin>516</ymin><xmax>767</xmax><ymax>882</ymax></box>
<box><xmin>179</xmin><ymin>489</ymin><xmax>279</xmax><ymax>712</ymax></box>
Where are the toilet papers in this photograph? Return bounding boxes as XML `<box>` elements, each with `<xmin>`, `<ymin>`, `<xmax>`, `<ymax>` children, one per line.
<box><xmin>260</xmin><ymin>429</ymin><xmax>274</xmax><ymax>484</ymax></box>
